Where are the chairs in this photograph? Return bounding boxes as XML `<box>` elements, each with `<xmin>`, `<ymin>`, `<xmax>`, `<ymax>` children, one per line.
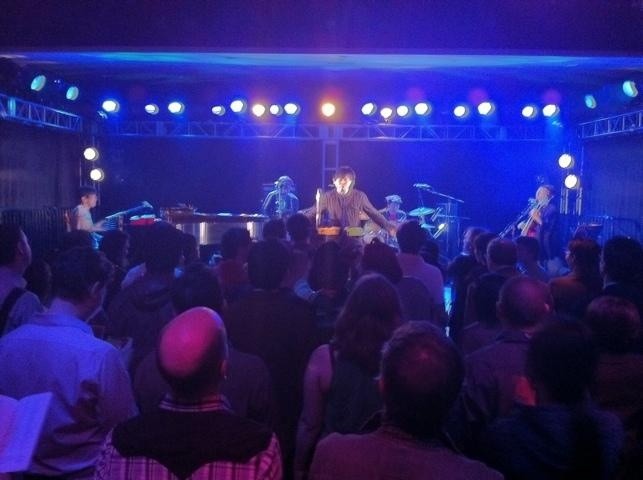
<box><xmin>63</xmin><ymin>211</ymin><xmax>71</xmax><ymax>235</ymax></box>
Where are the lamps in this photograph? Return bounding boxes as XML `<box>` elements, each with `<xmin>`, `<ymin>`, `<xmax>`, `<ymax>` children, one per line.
<box><xmin>21</xmin><ymin>63</ymin><xmax>46</xmax><ymax>93</ymax></box>
<box><xmin>50</xmin><ymin>75</ymin><xmax>78</xmax><ymax>102</ymax></box>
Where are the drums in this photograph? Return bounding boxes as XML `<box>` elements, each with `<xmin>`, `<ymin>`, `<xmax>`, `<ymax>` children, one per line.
<box><xmin>363</xmin><ymin>219</ymin><xmax>388</xmax><ymax>244</ymax></box>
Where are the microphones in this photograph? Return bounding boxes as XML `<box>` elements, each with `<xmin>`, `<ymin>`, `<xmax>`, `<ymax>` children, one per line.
<box><xmin>413</xmin><ymin>183</ymin><xmax>432</xmax><ymax>190</ymax></box>
<box><xmin>340</xmin><ymin>185</ymin><xmax>344</xmax><ymax>193</ymax></box>
<box><xmin>142</xmin><ymin>200</ymin><xmax>153</xmax><ymax>210</ymax></box>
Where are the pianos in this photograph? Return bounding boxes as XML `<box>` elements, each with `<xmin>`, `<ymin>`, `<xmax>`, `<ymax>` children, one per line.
<box><xmin>164</xmin><ymin>207</ymin><xmax>269</xmax><ymax>258</ymax></box>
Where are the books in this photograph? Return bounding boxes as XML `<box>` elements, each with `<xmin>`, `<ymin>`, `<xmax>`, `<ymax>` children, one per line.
<box><xmin>0</xmin><ymin>391</ymin><xmax>53</xmax><ymax>472</ymax></box>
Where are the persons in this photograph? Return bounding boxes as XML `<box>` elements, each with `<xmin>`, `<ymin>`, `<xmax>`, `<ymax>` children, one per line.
<box><xmin>360</xmin><ymin>242</ymin><xmax>436</xmax><ymax>322</ymax></box>
<box><xmin>443</xmin><ymin>226</ymin><xmax>487</xmax><ymax>344</ymax></box>
<box><xmin>0</xmin><ymin>244</ymin><xmax>138</xmax><ymax>479</ymax></box>
<box><xmin>585</xmin><ymin>290</ymin><xmax>643</xmax><ymax>479</ymax></box>
<box><xmin>376</xmin><ymin>196</ymin><xmax>406</xmax><ymax>222</ymax></box>
<box><xmin>99</xmin><ymin>229</ymin><xmax>129</xmax><ymax>322</ymax></box>
<box><xmin>281</xmin><ymin>242</ymin><xmax>317</xmax><ymax>299</ymax></box>
<box><xmin>308</xmin><ymin>320</ymin><xmax>503</xmax><ymax>479</ymax></box>
<box><xmin>463</xmin><ymin>237</ymin><xmax>521</xmax><ymax>332</ymax></box>
<box><xmin>578</xmin><ymin>237</ymin><xmax>643</xmax><ymax>320</ymax></box>
<box><xmin>452</xmin><ymin>274</ymin><xmax>553</xmax><ymax>450</ymax></box>
<box><xmin>472</xmin><ymin>317</ymin><xmax>624</xmax><ymax>479</ymax></box>
<box><xmin>515</xmin><ymin>235</ymin><xmax>551</xmax><ymax>287</ymax></box>
<box><xmin>293</xmin><ymin>274</ymin><xmax>410</xmax><ymax>479</ymax></box>
<box><xmin>227</xmin><ymin>241</ymin><xmax>317</xmax><ymax>480</ymax></box>
<box><xmin>91</xmin><ymin>304</ymin><xmax>284</xmax><ymax>480</ymax></box>
<box><xmin>511</xmin><ymin>184</ymin><xmax>560</xmax><ymax>260</ymax></box>
<box><xmin>60</xmin><ymin>231</ymin><xmax>95</xmax><ymax>250</ymax></box>
<box><xmin>587</xmin><ymin>241</ymin><xmax>606</xmax><ymax>285</ymax></box>
<box><xmin>1</xmin><ymin>223</ymin><xmax>41</xmax><ymax>335</ymax></box>
<box><xmin>286</xmin><ymin>212</ymin><xmax>317</xmax><ymax>264</ymax></box>
<box><xmin>132</xmin><ymin>261</ymin><xmax>275</xmax><ymax>425</ymax></box>
<box><xmin>306</xmin><ymin>259</ymin><xmax>351</xmax><ymax>341</ymax></box>
<box><xmin>25</xmin><ymin>261</ymin><xmax>53</xmax><ymax>296</ymax></box>
<box><xmin>462</xmin><ymin>232</ymin><xmax>504</xmax><ymax>306</ymax></box>
<box><xmin>292</xmin><ymin>241</ymin><xmax>358</xmax><ymax>363</ymax></box>
<box><xmin>213</xmin><ymin>228</ymin><xmax>253</xmax><ymax>298</ymax></box>
<box><xmin>418</xmin><ymin>242</ymin><xmax>446</xmax><ymax>285</ymax></box>
<box><xmin>393</xmin><ymin>220</ymin><xmax>449</xmax><ymax>327</ymax></box>
<box><xmin>108</xmin><ymin>222</ymin><xmax>186</xmax><ymax>361</ymax></box>
<box><xmin>260</xmin><ymin>220</ymin><xmax>285</xmax><ymax>241</ymax></box>
<box><xmin>550</xmin><ymin>239</ymin><xmax>593</xmax><ymax>303</ymax></box>
<box><xmin>303</xmin><ymin>166</ymin><xmax>398</xmax><ymax>243</ymax></box>
<box><xmin>173</xmin><ymin>233</ymin><xmax>201</xmax><ymax>263</ymax></box>
<box><xmin>68</xmin><ymin>186</ymin><xmax>97</xmax><ymax>229</ymax></box>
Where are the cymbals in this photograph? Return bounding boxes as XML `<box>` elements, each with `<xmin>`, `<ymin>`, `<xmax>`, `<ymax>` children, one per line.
<box><xmin>409</xmin><ymin>207</ymin><xmax>434</xmax><ymax>217</ymax></box>
<box><xmin>421</xmin><ymin>223</ymin><xmax>435</xmax><ymax>229</ymax></box>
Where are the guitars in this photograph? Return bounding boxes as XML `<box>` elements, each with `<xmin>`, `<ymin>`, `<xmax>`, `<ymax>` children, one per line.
<box><xmin>520</xmin><ymin>196</ymin><xmax>549</xmax><ymax>236</ymax></box>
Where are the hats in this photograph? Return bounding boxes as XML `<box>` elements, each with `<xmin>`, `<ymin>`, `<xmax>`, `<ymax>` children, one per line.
<box><xmin>386</xmin><ymin>196</ymin><xmax>402</xmax><ymax>204</ymax></box>
